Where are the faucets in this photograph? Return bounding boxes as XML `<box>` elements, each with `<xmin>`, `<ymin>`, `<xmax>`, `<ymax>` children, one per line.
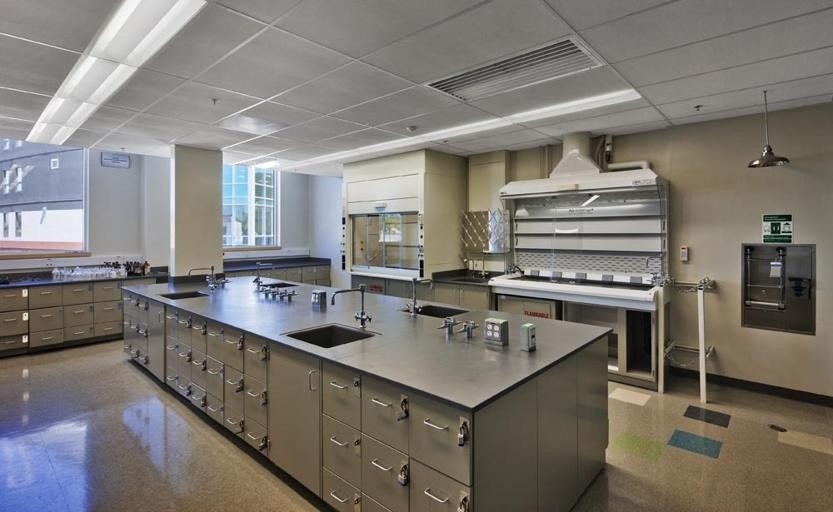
<box><xmin>464</xmin><ymin>259</ymin><xmax>477</xmax><ymax>279</ymax></box>
<box><xmin>407</xmin><ymin>278</ymin><xmax>434</xmax><ymax>316</ymax></box>
<box><xmin>187</xmin><ymin>266</ymin><xmax>219</xmax><ymax>291</ymax></box>
<box><xmin>331</xmin><ymin>284</ymin><xmax>372</xmax><ymax>328</ymax></box>
<box><xmin>252</xmin><ymin>261</ymin><xmax>274</xmax><ymax>287</ymax></box>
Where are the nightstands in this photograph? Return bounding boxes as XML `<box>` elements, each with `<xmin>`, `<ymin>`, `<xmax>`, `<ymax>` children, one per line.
<box><xmin>166</xmin><ymin>304</ymin><xmax>267</xmax><ymax>458</ymax></box>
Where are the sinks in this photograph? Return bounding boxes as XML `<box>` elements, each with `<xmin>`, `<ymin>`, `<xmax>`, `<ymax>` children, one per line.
<box><xmin>159</xmin><ymin>290</ymin><xmax>211</xmax><ymax>301</ymax></box>
<box><xmin>259</xmin><ymin>281</ymin><xmax>301</xmax><ymax>290</ymax></box>
<box><xmin>284</xmin><ymin>322</ymin><xmax>381</xmax><ymax>349</ymax></box>
<box><xmin>401</xmin><ymin>305</ymin><xmax>471</xmax><ymax>320</ymax></box>
<box><xmin>453</xmin><ymin>277</ymin><xmax>490</xmax><ymax>283</ymax></box>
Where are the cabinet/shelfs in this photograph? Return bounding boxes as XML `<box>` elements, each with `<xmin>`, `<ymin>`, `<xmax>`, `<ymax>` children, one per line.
<box><xmin>432</xmin><ymin>282</ymin><xmax>496</xmax><ymax>311</ymax></box>
<box><xmin>0</xmin><ymin>278</ymin><xmax>156</xmax><ymax>358</ymax></box>
<box><xmin>497</xmin><ymin>295</ymin><xmax>556</xmax><ymax>321</ymax></box>
<box><xmin>267</xmin><ymin>339</ymin><xmax>322</xmax><ymax>500</ymax></box>
<box><xmin>147</xmin><ymin>299</ymin><xmax>165</xmax><ymax>386</ymax></box>
<box><xmin>386</xmin><ymin>280</ymin><xmax>434</xmax><ymax>303</ymax></box>
<box><xmin>350</xmin><ymin>274</ymin><xmax>385</xmax><ymax>295</ymax></box>
<box><xmin>224</xmin><ymin>265</ymin><xmax>330</xmax><ymax>289</ymax></box>
<box><xmin>320</xmin><ymin>334</ymin><xmax>608</xmax><ymax>512</ymax></box>
<box><xmin>123</xmin><ymin>291</ymin><xmax>150</xmax><ymax>371</ymax></box>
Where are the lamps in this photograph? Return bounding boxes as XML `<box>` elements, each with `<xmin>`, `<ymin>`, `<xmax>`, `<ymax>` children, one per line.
<box><xmin>747</xmin><ymin>89</ymin><xmax>790</xmax><ymax>172</ymax></box>
